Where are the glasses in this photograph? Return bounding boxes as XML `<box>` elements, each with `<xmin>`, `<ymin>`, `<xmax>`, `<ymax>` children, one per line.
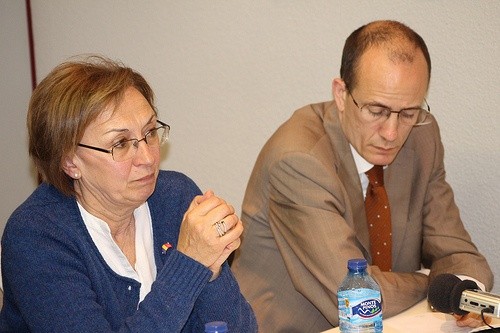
<box><xmin>343</xmin><ymin>79</ymin><xmax>430</xmax><ymax>125</ymax></box>
<box><xmin>78</xmin><ymin>119</ymin><xmax>170</xmax><ymax>162</ymax></box>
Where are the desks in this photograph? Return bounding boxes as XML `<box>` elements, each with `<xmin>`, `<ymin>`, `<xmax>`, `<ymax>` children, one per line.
<box><xmin>320</xmin><ymin>295</ymin><xmax>500</xmax><ymax>333</ymax></box>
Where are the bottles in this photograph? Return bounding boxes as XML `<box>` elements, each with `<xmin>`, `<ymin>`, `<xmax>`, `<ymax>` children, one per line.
<box><xmin>204</xmin><ymin>321</ymin><xmax>228</xmax><ymax>333</ymax></box>
<box><xmin>337</xmin><ymin>258</ymin><xmax>383</xmax><ymax>333</ymax></box>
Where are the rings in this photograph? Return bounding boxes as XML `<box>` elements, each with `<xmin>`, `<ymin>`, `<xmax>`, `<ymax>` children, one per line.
<box><xmin>215</xmin><ymin>220</ymin><xmax>227</xmax><ymax>236</ymax></box>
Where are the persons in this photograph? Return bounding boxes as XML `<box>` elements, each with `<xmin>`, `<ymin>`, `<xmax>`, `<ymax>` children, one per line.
<box><xmin>0</xmin><ymin>52</ymin><xmax>258</xmax><ymax>333</ymax></box>
<box><xmin>232</xmin><ymin>20</ymin><xmax>495</xmax><ymax>333</ymax></box>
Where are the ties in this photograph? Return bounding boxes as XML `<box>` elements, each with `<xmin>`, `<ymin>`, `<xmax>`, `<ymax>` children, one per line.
<box><xmin>363</xmin><ymin>165</ymin><xmax>392</xmax><ymax>273</ymax></box>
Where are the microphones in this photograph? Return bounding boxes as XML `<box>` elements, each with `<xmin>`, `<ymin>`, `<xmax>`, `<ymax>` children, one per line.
<box><xmin>427</xmin><ymin>273</ymin><xmax>500</xmax><ymax>319</ymax></box>
